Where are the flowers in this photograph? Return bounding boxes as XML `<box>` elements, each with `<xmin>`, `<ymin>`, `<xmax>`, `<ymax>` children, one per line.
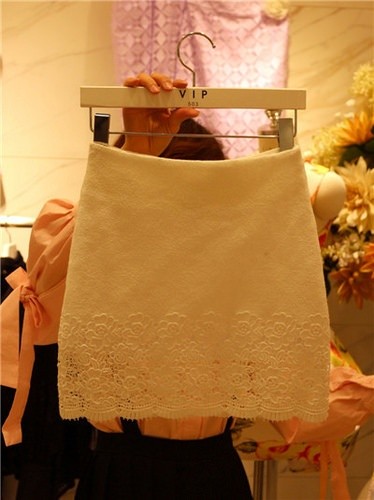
<box><xmin>310</xmin><ymin>62</ymin><xmax>374</xmax><ymax>312</ymax></box>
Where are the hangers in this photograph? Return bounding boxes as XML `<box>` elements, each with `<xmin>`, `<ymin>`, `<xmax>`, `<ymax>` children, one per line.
<box><xmin>79</xmin><ymin>29</ymin><xmax>307</xmax><ymax>153</ymax></box>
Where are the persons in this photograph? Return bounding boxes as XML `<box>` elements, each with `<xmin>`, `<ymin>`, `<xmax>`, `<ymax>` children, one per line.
<box><xmin>0</xmin><ymin>72</ymin><xmax>254</xmax><ymax>499</ymax></box>
